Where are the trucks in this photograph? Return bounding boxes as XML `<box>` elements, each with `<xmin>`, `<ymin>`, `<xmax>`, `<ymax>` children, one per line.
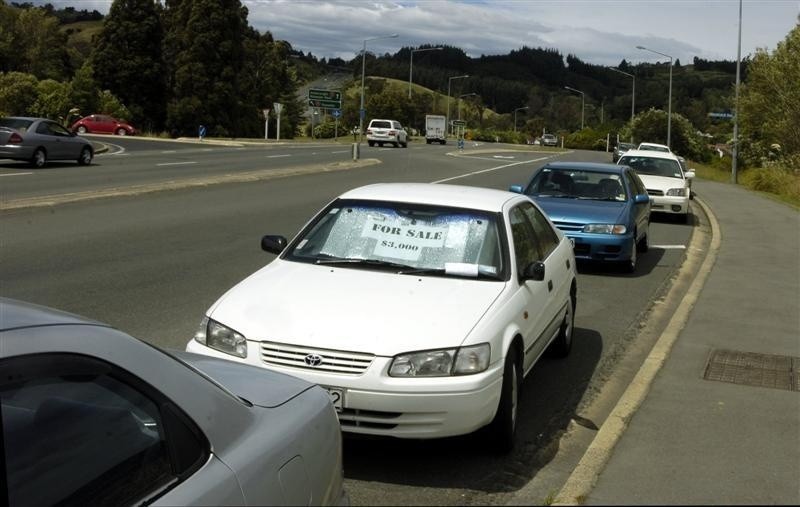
<box><xmin>425</xmin><ymin>114</ymin><xmax>449</xmax><ymax>145</ymax></box>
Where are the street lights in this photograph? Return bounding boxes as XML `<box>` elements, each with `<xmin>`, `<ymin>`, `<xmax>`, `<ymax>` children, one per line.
<box><xmin>635</xmin><ymin>45</ymin><xmax>673</xmax><ymax>150</ymax></box>
<box><xmin>514</xmin><ymin>105</ymin><xmax>529</xmax><ymax>132</ymax></box>
<box><xmin>408</xmin><ymin>47</ymin><xmax>444</xmax><ymax>99</ymax></box>
<box><xmin>457</xmin><ymin>92</ymin><xmax>477</xmax><ymax>120</ymax></box>
<box><xmin>609</xmin><ymin>66</ymin><xmax>636</xmax><ymax>127</ymax></box>
<box><xmin>564</xmin><ymin>85</ymin><xmax>585</xmax><ymax>130</ymax></box>
<box><xmin>446</xmin><ymin>74</ymin><xmax>470</xmax><ymax>139</ymax></box>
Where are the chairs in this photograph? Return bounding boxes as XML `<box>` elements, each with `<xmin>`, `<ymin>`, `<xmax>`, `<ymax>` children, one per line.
<box><xmin>631</xmin><ymin>159</ymin><xmax>672</xmax><ymax>176</ymax></box>
<box><xmin>8</xmin><ymin>397</ymin><xmax>157</xmax><ymax>503</ymax></box>
<box><xmin>551</xmin><ymin>174</ymin><xmax>622</xmax><ymax>202</ymax></box>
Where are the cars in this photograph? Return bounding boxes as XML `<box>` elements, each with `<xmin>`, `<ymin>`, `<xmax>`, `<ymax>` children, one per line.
<box><xmin>1</xmin><ymin>292</ymin><xmax>346</xmax><ymax>507</ymax></box>
<box><xmin>0</xmin><ymin>116</ymin><xmax>95</xmax><ymax>169</ymax></box>
<box><xmin>366</xmin><ymin>118</ymin><xmax>409</xmax><ymax>148</ymax></box>
<box><xmin>71</xmin><ymin>114</ymin><xmax>138</xmax><ymax>137</ymax></box>
<box><xmin>358</xmin><ymin>33</ymin><xmax>399</xmax><ymax>143</ymax></box>
<box><xmin>507</xmin><ymin>160</ymin><xmax>652</xmax><ymax>273</ymax></box>
<box><xmin>184</xmin><ymin>181</ymin><xmax>580</xmax><ymax>454</ymax></box>
<box><xmin>539</xmin><ymin>133</ymin><xmax>558</xmax><ymax>146</ymax></box>
<box><xmin>612</xmin><ymin>142</ymin><xmax>697</xmax><ymax>217</ymax></box>
